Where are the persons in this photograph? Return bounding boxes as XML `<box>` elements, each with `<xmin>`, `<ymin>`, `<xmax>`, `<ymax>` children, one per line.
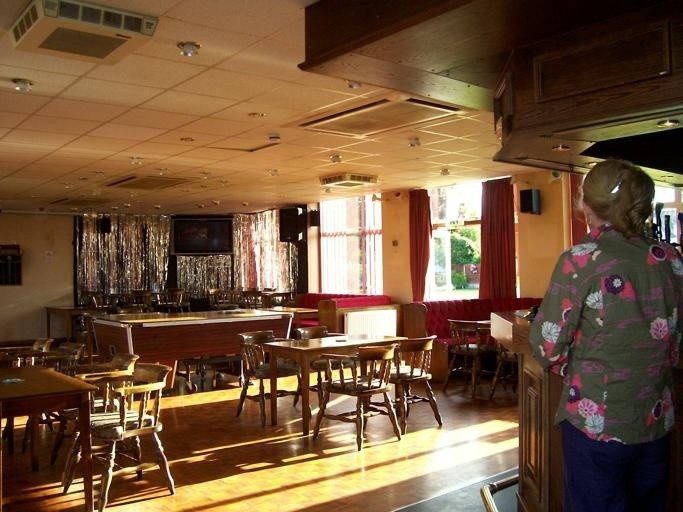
<box><xmin>527</xmin><ymin>157</ymin><xmax>681</xmax><ymax>511</ymax></box>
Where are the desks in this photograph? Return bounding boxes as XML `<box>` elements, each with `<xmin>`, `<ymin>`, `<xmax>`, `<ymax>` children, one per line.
<box><xmin>1</xmin><ymin>366</ymin><xmax>101</xmax><ymax>512</ymax></box>
<box><xmin>44</xmin><ymin>306</ymin><xmax>292</xmax><ymax>384</ymax></box>
<box><xmin>263</xmin><ymin>334</ymin><xmax>407</xmax><ymax>435</ymax></box>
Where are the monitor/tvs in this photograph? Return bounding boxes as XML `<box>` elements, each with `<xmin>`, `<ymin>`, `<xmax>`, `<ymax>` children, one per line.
<box><xmin>169</xmin><ymin>218</ymin><xmax>235</xmax><ymax>255</ymax></box>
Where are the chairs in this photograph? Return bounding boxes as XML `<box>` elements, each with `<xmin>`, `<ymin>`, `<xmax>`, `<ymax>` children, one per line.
<box><xmin>50</xmin><ymin>353</ymin><xmax>176</xmax><ymax>511</ymax></box>
<box><xmin>82</xmin><ymin>286</ymin><xmax>275</xmax><ymax>349</ymax></box>
<box><xmin>368</xmin><ymin>335</ymin><xmax>442</xmax><ymax>435</ymax></box>
<box><xmin>235</xmin><ymin>331</ymin><xmax>314</xmax><ymax>430</ymax></box>
<box><xmin>443</xmin><ymin>319</ymin><xmax>519</xmax><ymax>402</ymax></box>
<box><xmin>312</xmin><ymin>342</ymin><xmax>401</xmax><ymax>451</ymax></box>
<box><xmin>292</xmin><ymin>324</ymin><xmax>358</xmax><ymax>408</ymax></box>
<box><xmin>2</xmin><ymin>336</ymin><xmax>85</xmax><ymax>450</ymax></box>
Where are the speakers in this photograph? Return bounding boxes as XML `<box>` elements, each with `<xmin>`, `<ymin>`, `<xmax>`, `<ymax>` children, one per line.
<box><xmin>279</xmin><ymin>207</ymin><xmax>303</xmax><ymax>242</ymax></box>
<box><xmin>520</xmin><ymin>189</ymin><xmax>538</xmax><ymax>212</ymax></box>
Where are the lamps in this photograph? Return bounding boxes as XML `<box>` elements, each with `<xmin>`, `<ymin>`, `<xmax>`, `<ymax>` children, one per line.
<box><xmin>178</xmin><ymin>45</ymin><xmax>198</xmax><ymax>57</ymax></box>
<box><xmin>13</xmin><ymin>80</ymin><xmax>32</xmax><ymax>92</ymax></box>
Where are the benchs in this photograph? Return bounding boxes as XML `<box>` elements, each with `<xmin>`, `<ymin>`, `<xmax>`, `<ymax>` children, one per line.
<box><xmin>286</xmin><ymin>291</ymin><xmax>542</xmax><ymax>381</ymax></box>
